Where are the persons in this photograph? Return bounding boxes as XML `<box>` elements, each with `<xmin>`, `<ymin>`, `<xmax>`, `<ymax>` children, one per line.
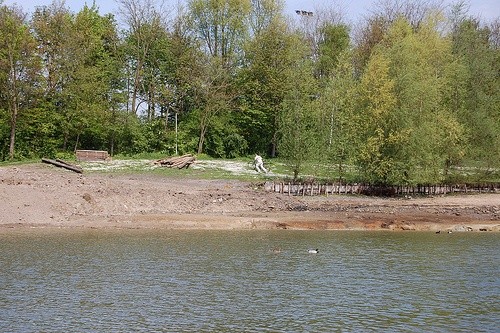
<box><xmin>254</xmin><ymin>151</ymin><xmax>268</xmax><ymax>175</ymax></box>
